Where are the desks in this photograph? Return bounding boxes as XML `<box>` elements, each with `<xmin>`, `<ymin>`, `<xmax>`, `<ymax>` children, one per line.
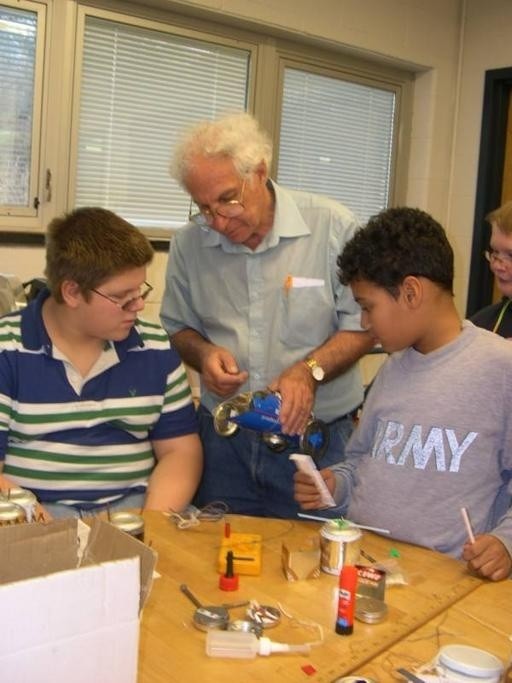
<box><xmin>98</xmin><ymin>507</ymin><xmax>511</xmax><ymax>683</ymax></box>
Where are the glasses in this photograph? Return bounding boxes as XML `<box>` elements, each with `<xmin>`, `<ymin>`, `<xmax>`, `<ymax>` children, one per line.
<box><xmin>73</xmin><ymin>275</ymin><xmax>155</xmax><ymax>310</ymax></box>
<box><xmin>483</xmin><ymin>249</ymin><xmax>511</xmax><ymax>270</ymax></box>
<box><xmin>186</xmin><ymin>170</ymin><xmax>249</xmax><ymax>226</ymax></box>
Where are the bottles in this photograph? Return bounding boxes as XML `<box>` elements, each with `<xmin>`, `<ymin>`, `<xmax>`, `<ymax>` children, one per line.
<box><xmin>440</xmin><ymin>644</ymin><xmax>503</xmax><ymax>682</ymax></box>
<box><xmin>205</xmin><ymin>630</ymin><xmax>310</xmax><ymax>659</ymax></box>
<box><xmin>319</xmin><ymin>523</ymin><xmax>363</xmax><ymax>577</ymax></box>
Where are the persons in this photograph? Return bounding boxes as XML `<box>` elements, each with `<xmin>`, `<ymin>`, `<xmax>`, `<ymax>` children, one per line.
<box><xmin>291</xmin><ymin>207</ymin><xmax>511</xmax><ymax>582</ymax></box>
<box><xmin>159</xmin><ymin>112</ymin><xmax>377</xmax><ymax>520</ymax></box>
<box><xmin>1</xmin><ymin>205</ymin><xmax>204</xmax><ymax>520</ymax></box>
<box><xmin>466</xmin><ymin>201</ymin><xmax>511</xmax><ymax>335</ymax></box>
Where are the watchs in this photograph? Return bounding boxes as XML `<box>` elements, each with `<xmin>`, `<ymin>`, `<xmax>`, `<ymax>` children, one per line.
<box><xmin>303</xmin><ymin>356</ymin><xmax>325</xmax><ymax>382</ymax></box>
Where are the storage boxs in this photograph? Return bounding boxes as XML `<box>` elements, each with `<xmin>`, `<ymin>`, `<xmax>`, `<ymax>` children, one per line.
<box><xmin>1</xmin><ymin>515</ymin><xmax>159</xmax><ymax>683</ymax></box>
